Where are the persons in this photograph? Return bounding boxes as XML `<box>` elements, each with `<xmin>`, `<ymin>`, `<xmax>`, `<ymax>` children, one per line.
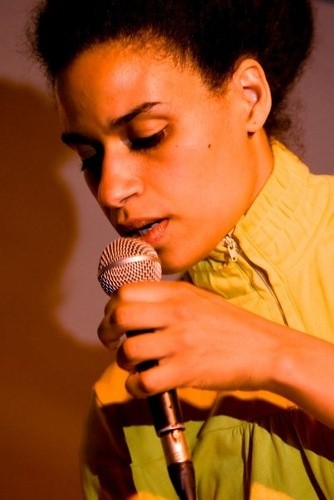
<box><xmin>24</xmin><ymin>0</ymin><xmax>334</xmax><ymax>500</ymax></box>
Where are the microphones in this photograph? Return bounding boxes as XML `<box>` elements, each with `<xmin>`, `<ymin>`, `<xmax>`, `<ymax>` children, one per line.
<box><xmin>98</xmin><ymin>238</ymin><xmax>198</xmax><ymax>499</ymax></box>
